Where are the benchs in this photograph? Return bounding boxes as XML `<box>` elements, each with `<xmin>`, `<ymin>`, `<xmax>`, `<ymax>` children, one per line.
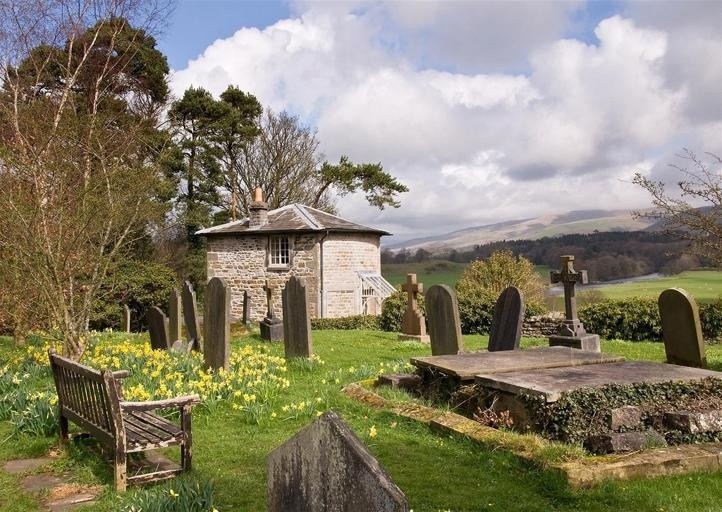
<box><xmin>48</xmin><ymin>347</ymin><xmax>200</xmax><ymax>493</ymax></box>
